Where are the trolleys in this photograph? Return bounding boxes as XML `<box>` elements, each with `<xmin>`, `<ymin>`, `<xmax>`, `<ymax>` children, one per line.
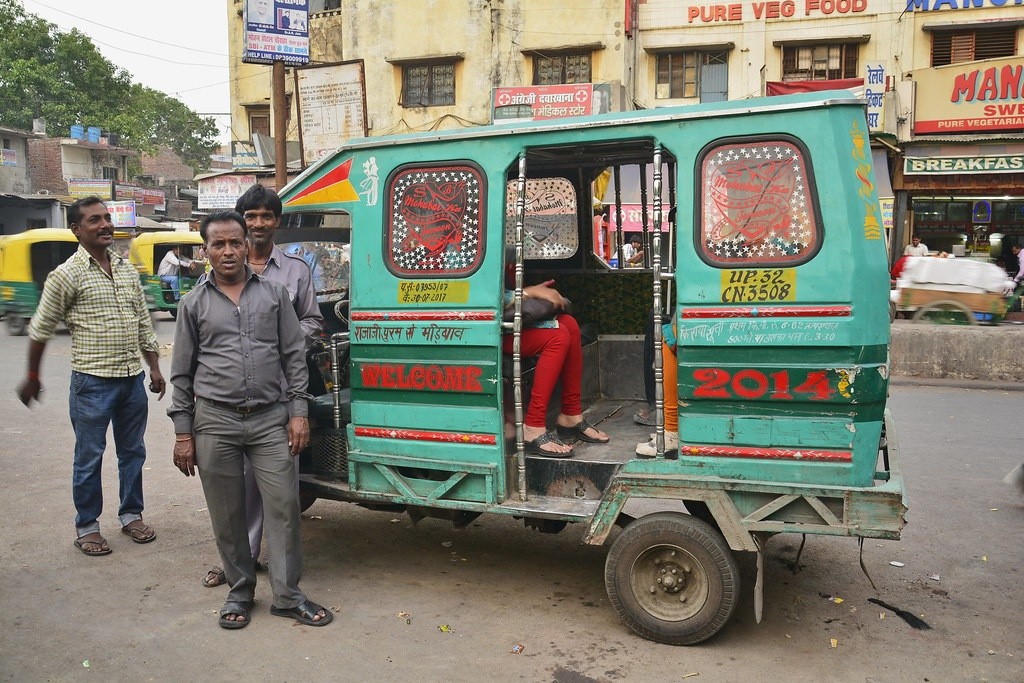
<box><xmin>895</xmin><ymin>281</ymin><xmax>1024</xmax><ymax>328</ymax></box>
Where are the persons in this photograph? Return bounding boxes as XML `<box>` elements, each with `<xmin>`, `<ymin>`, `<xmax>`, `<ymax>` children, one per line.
<box><xmin>157</xmin><ymin>242</ymin><xmax>207</xmax><ymax>301</ymax></box>
<box><xmin>502</xmin><ymin>255</ymin><xmax>609</xmax><ymax>458</ymax></box>
<box><xmin>316</xmin><ymin>245</ymin><xmax>349</xmax><ymax>291</ymax></box>
<box><xmin>166</xmin><ymin>210</ymin><xmax>333</xmax><ymax>629</ymax></box>
<box><xmin>903</xmin><ymin>233</ymin><xmax>928</xmax><ymax>257</ymax></box>
<box><xmin>632</xmin><ymin>307</ymin><xmax>678</xmax><ymax>460</ymax></box>
<box><xmin>612</xmin><ymin>235</ymin><xmax>643</xmax><ymax>268</ymax></box>
<box><xmin>195</xmin><ymin>184</ymin><xmax>324</xmax><ymax>588</ymax></box>
<box><xmin>1012</xmin><ymin>243</ymin><xmax>1024</xmax><ymax>291</ymax></box>
<box><xmin>16</xmin><ymin>195</ymin><xmax>166</xmax><ymax>556</ymax></box>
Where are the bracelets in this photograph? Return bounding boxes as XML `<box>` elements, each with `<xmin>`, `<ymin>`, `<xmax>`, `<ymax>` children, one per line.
<box><xmin>176</xmin><ymin>437</ymin><xmax>192</xmax><ymax>442</ymax></box>
<box><xmin>522</xmin><ymin>290</ymin><xmax>529</xmax><ymax>299</ymax></box>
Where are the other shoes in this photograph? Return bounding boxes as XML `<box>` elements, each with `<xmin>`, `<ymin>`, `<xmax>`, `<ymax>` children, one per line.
<box><xmin>633</xmin><ymin>408</ymin><xmax>658</xmax><ymax>426</ymax></box>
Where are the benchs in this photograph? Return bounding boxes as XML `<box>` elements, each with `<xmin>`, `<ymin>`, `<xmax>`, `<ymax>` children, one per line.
<box><xmin>309</xmin><ymin>386</ymin><xmax>352</xmax><ymax>423</ymax></box>
<box><xmin>502</xmin><ymin>319</ymin><xmax>601</xmax><ymax>458</ymax></box>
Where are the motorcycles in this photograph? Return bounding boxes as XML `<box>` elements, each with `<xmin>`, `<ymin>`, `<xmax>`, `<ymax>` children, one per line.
<box><xmin>196</xmin><ymin>87</ymin><xmax>908</xmax><ymax>646</ymax></box>
<box><xmin>128</xmin><ymin>230</ymin><xmax>213</xmax><ymax>321</ymax></box>
<box><xmin>0</xmin><ymin>226</ymin><xmax>131</xmax><ymax>337</ymax></box>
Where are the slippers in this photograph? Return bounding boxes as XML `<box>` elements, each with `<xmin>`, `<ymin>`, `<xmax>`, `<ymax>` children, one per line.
<box><xmin>74</xmin><ymin>538</ymin><xmax>112</xmax><ymax>556</ymax></box>
<box><xmin>203</xmin><ymin>559</ymin><xmax>262</xmax><ymax>588</ymax></box>
<box><xmin>121</xmin><ymin>519</ymin><xmax>156</xmax><ymax>544</ymax></box>
<box><xmin>525</xmin><ymin>429</ymin><xmax>575</xmax><ymax>458</ymax></box>
<box><xmin>555</xmin><ymin>415</ymin><xmax>610</xmax><ymax>443</ymax></box>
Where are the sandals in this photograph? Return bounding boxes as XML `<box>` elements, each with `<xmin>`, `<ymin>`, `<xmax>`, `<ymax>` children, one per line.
<box><xmin>270</xmin><ymin>598</ymin><xmax>334</xmax><ymax>627</ymax></box>
<box><xmin>219</xmin><ymin>602</ymin><xmax>254</xmax><ymax>629</ymax></box>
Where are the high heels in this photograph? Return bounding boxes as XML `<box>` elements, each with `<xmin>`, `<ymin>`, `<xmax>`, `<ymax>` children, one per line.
<box><xmin>636</xmin><ymin>431</ymin><xmax>679</xmax><ymax>459</ymax></box>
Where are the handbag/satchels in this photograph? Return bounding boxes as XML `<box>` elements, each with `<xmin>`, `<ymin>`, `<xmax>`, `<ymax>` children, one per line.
<box><xmin>503</xmin><ymin>293</ymin><xmax>572</xmax><ymax>327</ymax></box>
